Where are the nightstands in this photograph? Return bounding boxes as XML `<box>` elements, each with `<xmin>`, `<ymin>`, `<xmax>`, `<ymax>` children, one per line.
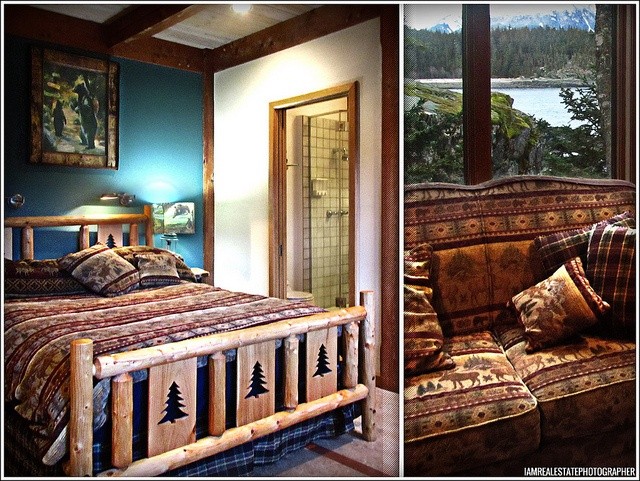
<box><xmin>189</xmin><ymin>267</ymin><xmax>209</xmax><ymax>283</ymax></box>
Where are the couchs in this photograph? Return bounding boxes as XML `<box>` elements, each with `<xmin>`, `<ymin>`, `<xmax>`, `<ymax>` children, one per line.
<box><xmin>404</xmin><ymin>175</ymin><xmax>636</xmax><ymax>477</ymax></box>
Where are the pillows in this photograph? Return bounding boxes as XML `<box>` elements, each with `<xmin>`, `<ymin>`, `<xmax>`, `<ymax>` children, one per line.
<box><xmin>506</xmin><ymin>255</ymin><xmax>611</xmax><ymax>355</ymax></box>
<box><xmin>404</xmin><ymin>243</ymin><xmax>458</xmax><ymax>374</ymax></box>
<box><xmin>582</xmin><ymin>220</ymin><xmax>636</xmax><ymax>333</ymax></box>
<box><xmin>56</xmin><ymin>242</ymin><xmax>141</xmax><ymax>297</ymax></box>
<box><xmin>531</xmin><ymin>209</ymin><xmax>636</xmax><ymax>278</ymax></box>
<box><xmin>131</xmin><ymin>251</ymin><xmax>183</xmax><ymax>288</ymax></box>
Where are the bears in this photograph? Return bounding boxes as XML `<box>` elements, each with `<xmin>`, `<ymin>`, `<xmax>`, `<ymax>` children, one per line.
<box><xmin>71</xmin><ymin>82</ymin><xmax>97</xmax><ymax>150</ymax></box>
<box><xmin>53</xmin><ymin>100</ymin><xmax>64</xmax><ymax>137</ymax></box>
<box><xmin>173</xmin><ymin>204</ymin><xmax>190</xmax><ymax>219</ymax></box>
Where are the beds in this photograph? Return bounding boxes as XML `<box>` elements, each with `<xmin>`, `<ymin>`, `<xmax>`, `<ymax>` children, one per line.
<box><xmin>5</xmin><ymin>206</ymin><xmax>377</xmax><ymax>477</ymax></box>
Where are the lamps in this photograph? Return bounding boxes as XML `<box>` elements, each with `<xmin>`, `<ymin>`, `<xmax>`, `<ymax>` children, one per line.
<box><xmin>152</xmin><ymin>202</ymin><xmax>196</xmax><ymax>251</ymax></box>
<box><xmin>97</xmin><ymin>191</ymin><xmax>135</xmax><ymax>206</ymax></box>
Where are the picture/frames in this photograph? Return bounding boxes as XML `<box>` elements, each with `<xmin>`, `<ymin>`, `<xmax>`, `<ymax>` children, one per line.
<box><xmin>27</xmin><ymin>44</ymin><xmax>121</xmax><ymax>171</ymax></box>
